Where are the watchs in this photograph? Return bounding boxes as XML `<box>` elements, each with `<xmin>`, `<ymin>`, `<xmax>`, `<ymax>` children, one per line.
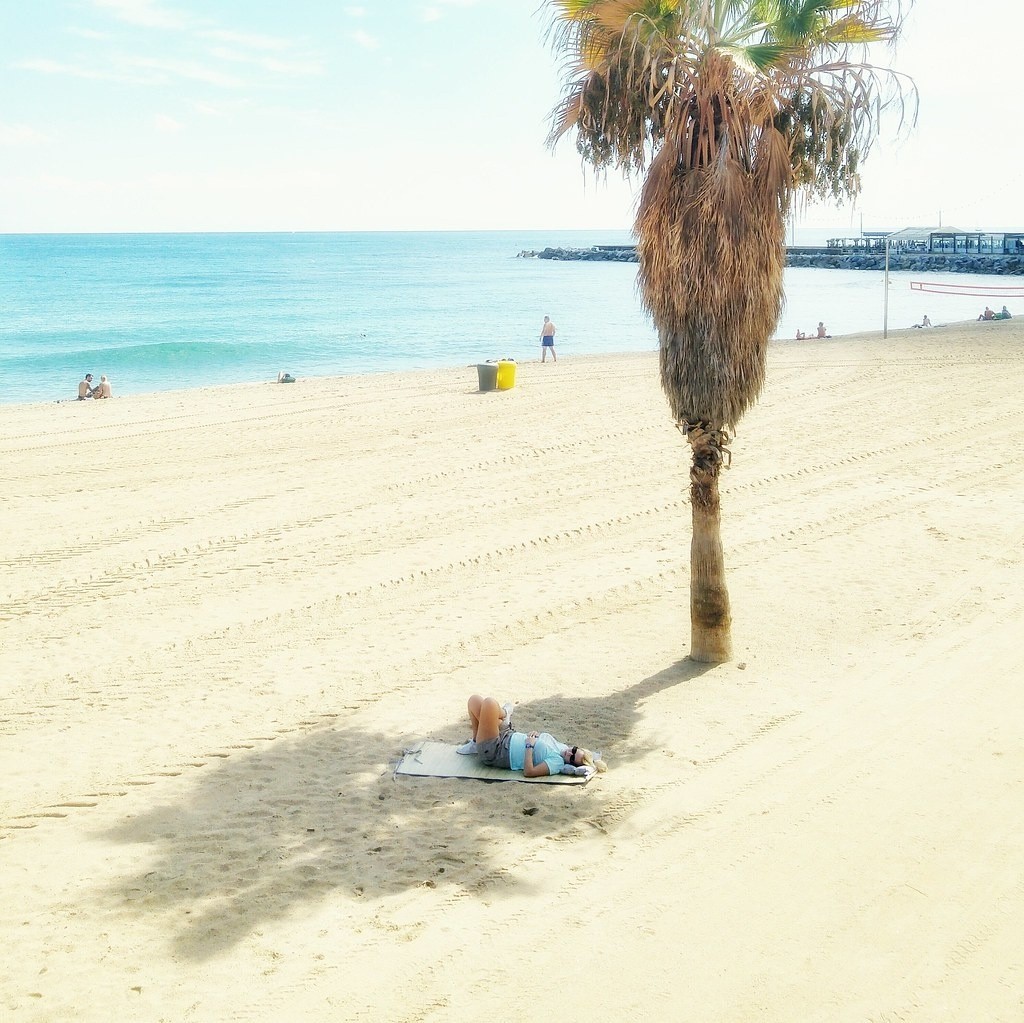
<box><xmin>525</xmin><ymin>744</ymin><xmax>533</xmax><ymax>748</ymax></box>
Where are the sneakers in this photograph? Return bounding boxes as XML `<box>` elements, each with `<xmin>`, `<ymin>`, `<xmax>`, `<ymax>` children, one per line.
<box><xmin>456</xmin><ymin>740</ymin><xmax>478</xmax><ymax>754</ymax></box>
<box><xmin>498</xmin><ymin>703</ymin><xmax>514</xmax><ymax>730</ymax></box>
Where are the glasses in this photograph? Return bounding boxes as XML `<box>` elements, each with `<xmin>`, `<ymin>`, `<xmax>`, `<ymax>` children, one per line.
<box><xmin>570</xmin><ymin>745</ymin><xmax>577</xmax><ymax>764</ymax></box>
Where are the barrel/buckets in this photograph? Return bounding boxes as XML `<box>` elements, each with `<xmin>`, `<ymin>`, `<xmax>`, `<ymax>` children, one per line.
<box><xmin>496</xmin><ymin>361</ymin><xmax>516</xmax><ymax>389</ymax></box>
<box><xmin>477</xmin><ymin>362</ymin><xmax>498</xmax><ymax>391</ymax></box>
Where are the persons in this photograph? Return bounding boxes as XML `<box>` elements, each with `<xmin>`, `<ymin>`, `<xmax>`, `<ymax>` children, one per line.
<box><xmin>797</xmin><ymin>329</ymin><xmax>805</xmax><ymax>340</ymax></box>
<box><xmin>277</xmin><ymin>372</ymin><xmax>290</xmax><ymax>382</ymax></box>
<box><xmin>1002</xmin><ymin>306</ymin><xmax>1011</xmax><ymax>318</ymax></box>
<box><xmin>540</xmin><ymin>316</ymin><xmax>556</xmax><ymax>362</ymax></box>
<box><xmin>977</xmin><ymin>307</ymin><xmax>995</xmax><ymax>321</ymax></box>
<box><xmin>913</xmin><ymin>315</ymin><xmax>931</xmax><ymax>328</ymax></box>
<box><xmin>817</xmin><ymin>322</ymin><xmax>826</xmax><ymax>338</ymax></box>
<box><xmin>78</xmin><ymin>374</ymin><xmax>112</xmax><ymax>399</ymax></box>
<box><xmin>458</xmin><ymin>695</ymin><xmax>608</xmax><ymax>777</ymax></box>
